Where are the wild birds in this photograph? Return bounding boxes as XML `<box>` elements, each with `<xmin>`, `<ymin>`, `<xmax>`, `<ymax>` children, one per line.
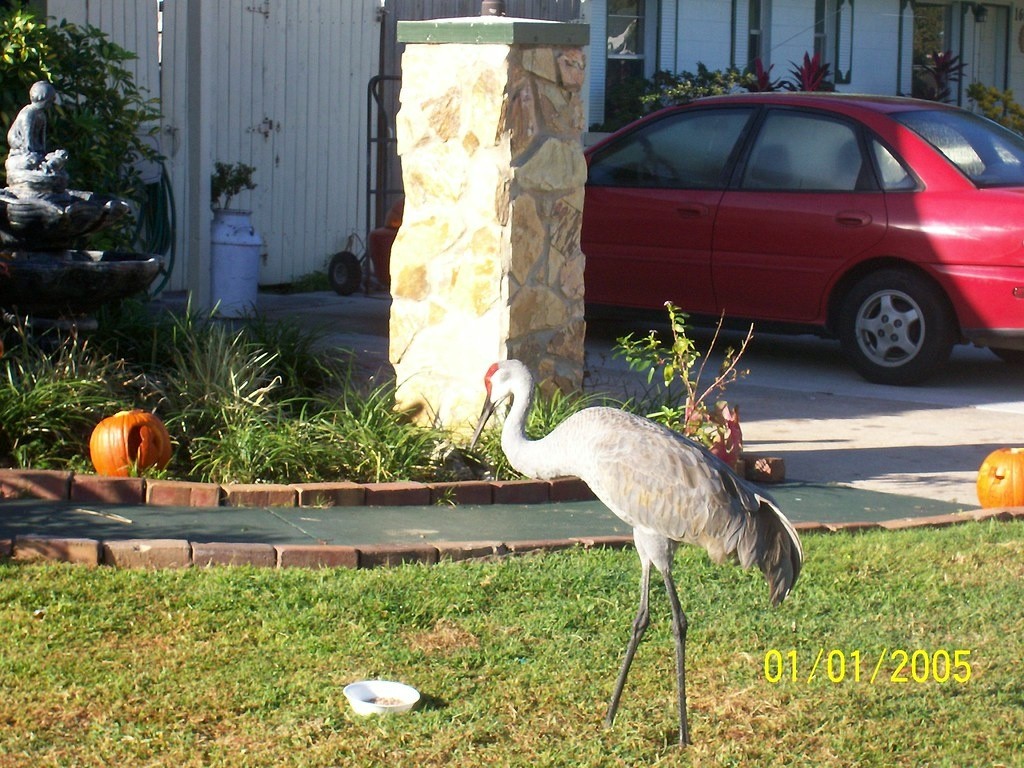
<box><xmin>469</xmin><ymin>359</ymin><xmax>804</xmax><ymax>747</ymax></box>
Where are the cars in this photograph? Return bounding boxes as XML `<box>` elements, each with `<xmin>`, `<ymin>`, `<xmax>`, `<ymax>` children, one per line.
<box><xmin>368</xmin><ymin>89</ymin><xmax>1024</xmax><ymax>390</ymax></box>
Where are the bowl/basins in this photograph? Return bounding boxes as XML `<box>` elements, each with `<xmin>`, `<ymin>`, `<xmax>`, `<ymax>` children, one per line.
<box><xmin>342</xmin><ymin>680</ymin><xmax>421</xmax><ymax>715</ymax></box>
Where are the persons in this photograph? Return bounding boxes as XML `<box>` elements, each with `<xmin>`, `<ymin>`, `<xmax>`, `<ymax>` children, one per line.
<box><xmin>5</xmin><ymin>80</ymin><xmax>69</xmax><ymax>172</ymax></box>
